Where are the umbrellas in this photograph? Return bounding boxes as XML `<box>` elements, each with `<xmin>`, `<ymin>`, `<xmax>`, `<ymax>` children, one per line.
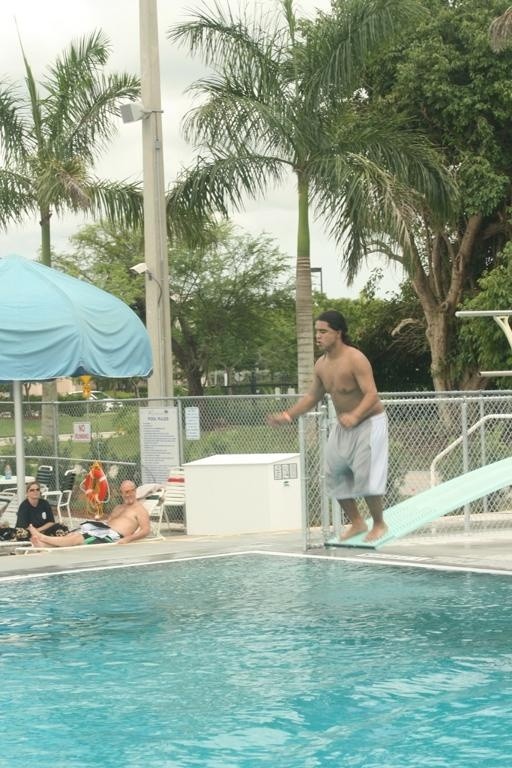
<box><xmin>0</xmin><ymin>253</ymin><xmax>154</xmax><ymax>501</ymax></box>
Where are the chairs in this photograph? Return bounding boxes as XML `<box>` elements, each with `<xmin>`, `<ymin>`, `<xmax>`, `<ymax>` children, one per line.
<box><xmin>1</xmin><ymin>466</ymin><xmax>183</xmax><ymax>555</ymax></box>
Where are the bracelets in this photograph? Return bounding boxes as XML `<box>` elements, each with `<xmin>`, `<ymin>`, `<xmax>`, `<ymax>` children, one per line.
<box><xmin>282</xmin><ymin>411</ymin><xmax>293</xmax><ymax>424</ymax></box>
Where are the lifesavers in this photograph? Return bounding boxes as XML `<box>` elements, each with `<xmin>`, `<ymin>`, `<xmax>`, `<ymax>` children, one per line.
<box><xmin>84</xmin><ymin>469</ymin><xmax>106</xmax><ymax>505</ymax></box>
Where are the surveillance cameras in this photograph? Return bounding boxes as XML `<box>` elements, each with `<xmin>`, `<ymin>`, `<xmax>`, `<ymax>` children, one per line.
<box><xmin>129</xmin><ymin>262</ymin><xmax>148</xmax><ymax>275</ymax></box>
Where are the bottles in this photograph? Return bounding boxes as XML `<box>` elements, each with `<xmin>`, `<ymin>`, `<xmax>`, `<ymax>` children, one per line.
<box><xmin>5</xmin><ymin>465</ymin><xmax>12</xmax><ymax>479</ymax></box>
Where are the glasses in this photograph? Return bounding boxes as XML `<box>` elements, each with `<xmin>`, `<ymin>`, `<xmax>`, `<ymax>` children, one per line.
<box><xmin>30</xmin><ymin>487</ymin><xmax>40</xmax><ymax>492</ymax></box>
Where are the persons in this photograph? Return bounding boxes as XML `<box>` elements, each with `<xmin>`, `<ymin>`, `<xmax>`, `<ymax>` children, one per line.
<box><xmin>268</xmin><ymin>310</ymin><xmax>389</xmax><ymax>542</ymax></box>
<box><xmin>27</xmin><ymin>479</ymin><xmax>151</xmax><ymax>547</ymax></box>
<box><xmin>14</xmin><ymin>483</ymin><xmax>69</xmax><ymax>541</ymax></box>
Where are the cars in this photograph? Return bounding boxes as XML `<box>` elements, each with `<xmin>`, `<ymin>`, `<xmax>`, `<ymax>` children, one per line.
<box><xmin>66</xmin><ymin>389</ymin><xmax>124</xmax><ymax>412</ymax></box>
